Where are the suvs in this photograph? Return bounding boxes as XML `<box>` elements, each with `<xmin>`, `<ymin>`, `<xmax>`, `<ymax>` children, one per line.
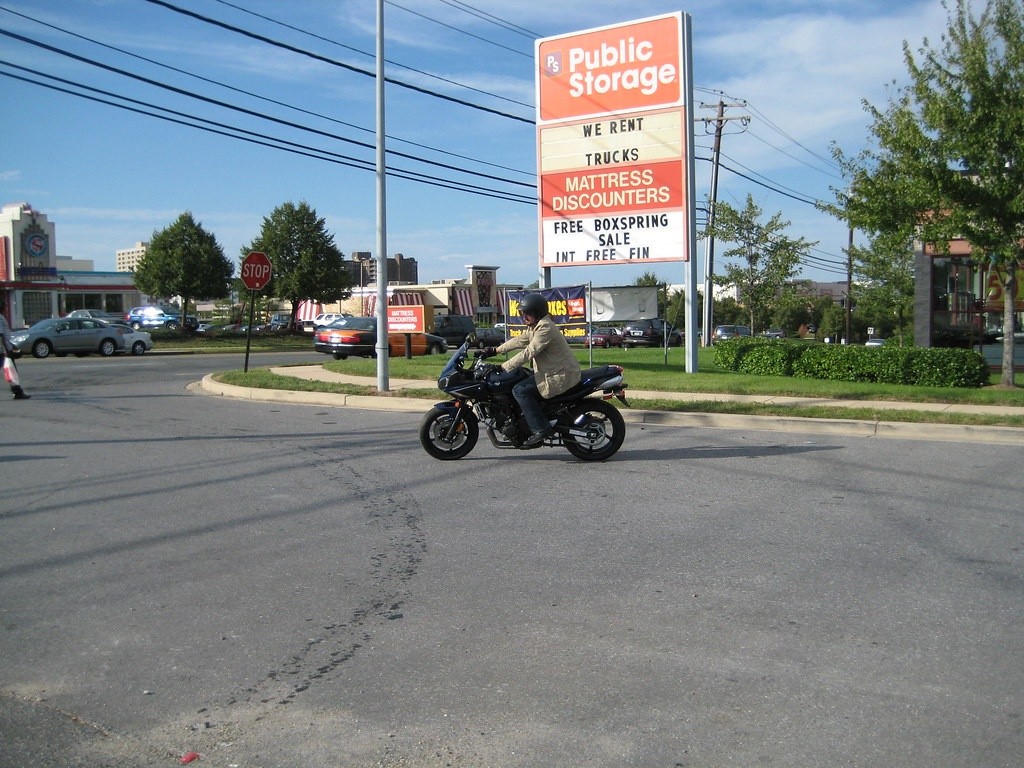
<box><xmin>125</xmin><ymin>305</ymin><xmax>200</xmax><ymax>331</ymax></box>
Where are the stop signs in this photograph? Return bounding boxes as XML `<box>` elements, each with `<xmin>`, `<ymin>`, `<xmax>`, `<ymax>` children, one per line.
<box><xmin>241</xmin><ymin>251</ymin><xmax>272</xmax><ymax>290</ymax></box>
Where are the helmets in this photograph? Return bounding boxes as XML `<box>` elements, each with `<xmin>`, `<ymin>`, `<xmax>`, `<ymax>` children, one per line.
<box><xmin>517</xmin><ymin>294</ymin><xmax>548</xmax><ymax>322</ymax></box>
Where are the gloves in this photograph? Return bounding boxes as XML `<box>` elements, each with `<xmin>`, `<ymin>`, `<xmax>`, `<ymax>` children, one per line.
<box><xmin>484</xmin><ymin>363</ymin><xmax>503</xmax><ymax>373</ymax></box>
<box><xmin>482</xmin><ymin>346</ymin><xmax>496</xmax><ymax>359</ymax></box>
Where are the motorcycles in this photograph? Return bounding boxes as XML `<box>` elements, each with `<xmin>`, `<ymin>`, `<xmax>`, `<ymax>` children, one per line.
<box><xmin>419</xmin><ymin>330</ymin><xmax>631</xmax><ymax>462</ymax></box>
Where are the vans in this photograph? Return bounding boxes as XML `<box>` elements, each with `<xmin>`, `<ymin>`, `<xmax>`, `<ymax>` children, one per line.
<box><xmin>434</xmin><ymin>314</ymin><xmax>476</xmax><ymax>349</ymax></box>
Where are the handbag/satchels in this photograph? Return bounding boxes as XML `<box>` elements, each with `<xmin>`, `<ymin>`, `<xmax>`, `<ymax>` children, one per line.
<box><xmin>5</xmin><ymin>359</ymin><xmax>19</xmax><ymax>386</ymax></box>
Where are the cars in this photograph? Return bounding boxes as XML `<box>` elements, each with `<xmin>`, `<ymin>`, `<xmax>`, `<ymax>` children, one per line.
<box><xmin>865</xmin><ymin>339</ymin><xmax>885</xmax><ymax>347</ymax></box>
<box><xmin>195</xmin><ymin>313</ymin><xmax>355</xmax><ymax>332</ymax></box>
<box><xmin>314</xmin><ymin>316</ymin><xmax>448</xmax><ymax>361</ymax></box>
<box><xmin>585</xmin><ymin>318</ymin><xmax>701</xmax><ymax>349</ymax></box>
<box><xmin>465</xmin><ymin>328</ymin><xmax>506</xmax><ymax>349</ymax></box>
<box><xmin>712</xmin><ymin>325</ymin><xmax>751</xmax><ymax>347</ymax></box>
<box><xmin>9</xmin><ymin>318</ymin><xmax>126</xmax><ymax>359</ymax></box>
<box><xmin>64</xmin><ymin>309</ymin><xmax>126</xmax><ymax>329</ymax></box>
<box><xmin>760</xmin><ymin>329</ymin><xmax>785</xmax><ymax>338</ymax></box>
<box><xmin>107</xmin><ymin>324</ymin><xmax>154</xmax><ymax>355</ymax></box>
<box><xmin>494</xmin><ymin>323</ymin><xmax>505</xmax><ymax>328</ymax></box>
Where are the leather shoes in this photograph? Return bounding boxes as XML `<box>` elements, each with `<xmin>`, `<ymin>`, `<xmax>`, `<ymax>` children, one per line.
<box><xmin>523</xmin><ymin>427</ymin><xmax>556</xmax><ymax>448</ymax></box>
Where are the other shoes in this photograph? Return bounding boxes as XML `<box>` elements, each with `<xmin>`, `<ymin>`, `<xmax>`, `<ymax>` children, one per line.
<box><xmin>13</xmin><ymin>394</ymin><xmax>31</xmax><ymax>399</ymax></box>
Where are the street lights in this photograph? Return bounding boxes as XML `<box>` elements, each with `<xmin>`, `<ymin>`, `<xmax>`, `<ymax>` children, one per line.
<box><xmin>352</xmin><ymin>252</ymin><xmax>372</xmax><ymax>316</ymax></box>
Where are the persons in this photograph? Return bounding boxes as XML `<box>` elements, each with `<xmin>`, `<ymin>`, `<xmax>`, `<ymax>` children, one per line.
<box><xmin>481</xmin><ymin>293</ymin><xmax>581</xmax><ymax>446</ymax></box>
<box><xmin>0</xmin><ymin>299</ymin><xmax>31</xmax><ymax>400</ymax></box>
